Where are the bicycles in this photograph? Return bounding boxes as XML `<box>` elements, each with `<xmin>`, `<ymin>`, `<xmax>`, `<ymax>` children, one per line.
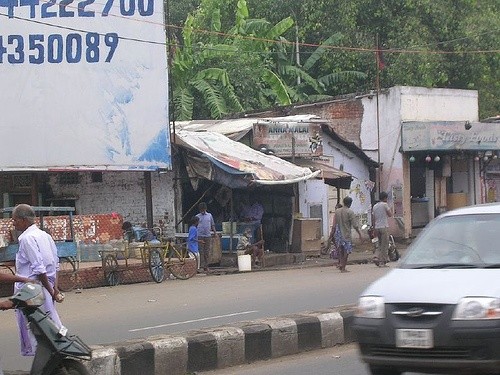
<box><xmin>57</xmin><ymin>252</ymin><xmax>81</xmax><ymax>291</ymax></box>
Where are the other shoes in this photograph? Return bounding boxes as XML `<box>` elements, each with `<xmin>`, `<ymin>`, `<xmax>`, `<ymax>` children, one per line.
<box><xmin>374</xmin><ymin>259</ymin><xmax>379</xmax><ymax>266</ymax></box>
<box><xmin>383</xmin><ymin>264</ymin><xmax>389</xmax><ymax>267</ymax></box>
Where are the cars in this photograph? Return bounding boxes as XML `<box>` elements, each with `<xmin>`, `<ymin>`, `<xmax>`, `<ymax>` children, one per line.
<box><xmin>351</xmin><ymin>202</ymin><xmax>500</xmax><ymax>375</ymax></box>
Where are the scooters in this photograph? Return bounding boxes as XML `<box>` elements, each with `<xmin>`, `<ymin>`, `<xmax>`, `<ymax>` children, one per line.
<box><xmin>361</xmin><ymin>218</ymin><xmax>399</xmax><ymax>264</ymax></box>
<box><xmin>0</xmin><ymin>281</ymin><xmax>94</xmax><ymax>375</ymax></box>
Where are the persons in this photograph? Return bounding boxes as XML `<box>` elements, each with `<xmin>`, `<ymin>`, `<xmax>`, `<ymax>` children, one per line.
<box><xmin>188</xmin><ymin>216</ymin><xmax>206</xmax><ymax>273</ymax></box>
<box><xmin>0</xmin><ymin>273</ymin><xmax>41</xmax><ymax>310</ymax></box>
<box><xmin>236</xmin><ymin>227</ymin><xmax>265</xmax><ymax>268</ymax></box>
<box><xmin>122</xmin><ymin>221</ymin><xmax>163</xmax><ymax>282</ymax></box>
<box><xmin>335</xmin><ymin>197</ymin><xmax>363</xmax><ymax>272</ymax></box>
<box><xmin>330</xmin><ymin>204</ymin><xmax>343</xmax><ymax>268</ymax></box>
<box><xmin>196</xmin><ymin>203</ymin><xmax>218</xmax><ymax>273</ymax></box>
<box><xmin>235</xmin><ymin>191</ymin><xmax>264</xmax><ymax>244</ymax></box>
<box><xmin>12</xmin><ymin>204</ymin><xmax>65</xmax><ymax>356</ymax></box>
<box><xmin>371</xmin><ymin>192</ymin><xmax>393</xmax><ymax>266</ymax></box>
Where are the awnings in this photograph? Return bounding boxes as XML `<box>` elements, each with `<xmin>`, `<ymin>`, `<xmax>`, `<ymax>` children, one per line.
<box><xmin>177</xmin><ymin>131</ymin><xmax>323</xmax><ymax>190</ymax></box>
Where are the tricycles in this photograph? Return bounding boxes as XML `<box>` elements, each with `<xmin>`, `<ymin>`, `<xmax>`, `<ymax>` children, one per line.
<box><xmin>102</xmin><ymin>236</ymin><xmax>199</xmax><ymax>287</ymax></box>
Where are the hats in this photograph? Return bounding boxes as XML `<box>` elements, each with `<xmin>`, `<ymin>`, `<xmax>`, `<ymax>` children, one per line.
<box><xmin>245</xmin><ymin>228</ymin><xmax>253</xmax><ymax>237</ymax></box>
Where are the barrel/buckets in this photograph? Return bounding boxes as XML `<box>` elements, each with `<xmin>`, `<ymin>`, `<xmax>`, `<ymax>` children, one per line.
<box><xmin>222</xmin><ymin>221</ymin><xmax>237</xmax><ymax>234</ymax></box>
<box><xmin>238</xmin><ymin>255</ymin><xmax>252</xmax><ymax>272</ymax></box>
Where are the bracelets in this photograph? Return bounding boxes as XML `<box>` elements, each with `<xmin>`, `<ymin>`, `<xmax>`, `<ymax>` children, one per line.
<box><xmin>250</xmin><ymin>218</ymin><xmax>253</xmax><ymax>221</ymax></box>
